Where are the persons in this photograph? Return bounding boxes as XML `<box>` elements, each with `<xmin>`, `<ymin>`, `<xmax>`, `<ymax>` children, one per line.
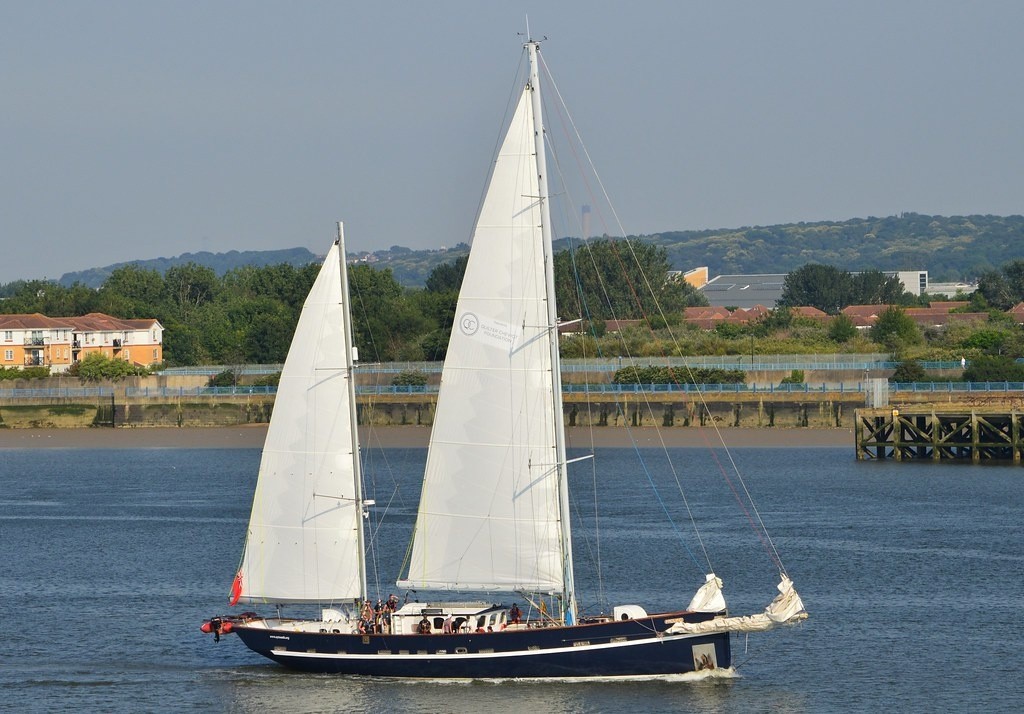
<box><xmin>419</xmin><ymin>614</ymin><xmax>432</xmax><ymax>634</ymax></box>
<box><xmin>442</xmin><ymin>615</ymin><xmax>453</xmax><ymax>634</ymax></box>
<box><xmin>488</xmin><ymin>626</ymin><xmax>494</xmax><ymax>632</ymax></box>
<box><xmin>476</xmin><ymin>627</ymin><xmax>485</xmax><ymax>633</ymax></box>
<box><xmin>510</xmin><ymin>603</ymin><xmax>522</xmax><ymax>623</ymax></box>
<box><xmin>359</xmin><ymin>594</ymin><xmax>396</xmax><ymax>633</ymax></box>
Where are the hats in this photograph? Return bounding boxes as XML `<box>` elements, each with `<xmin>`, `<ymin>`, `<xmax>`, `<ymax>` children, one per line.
<box><xmin>365</xmin><ymin>600</ymin><xmax>372</xmax><ymax>603</ymax></box>
<box><xmin>512</xmin><ymin>603</ymin><xmax>517</xmax><ymax>607</ymax></box>
<box><xmin>446</xmin><ymin>614</ymin><xmax>452</xmax><ymax>619</ymax></box>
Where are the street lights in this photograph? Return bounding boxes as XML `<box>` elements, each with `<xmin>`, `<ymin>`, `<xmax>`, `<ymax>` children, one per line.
<box><xmin>750</xmin><ymin>334</ymin><xmax>754</xmax><ymax>369</ymax></box>
<box><xmin>866</xmin><ymin>369</ymin><xmax>870</xmax><ymax>392</ymax></box>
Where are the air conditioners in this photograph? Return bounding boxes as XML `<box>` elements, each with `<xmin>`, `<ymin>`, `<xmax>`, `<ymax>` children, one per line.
<box><xmin>73</xmin><ymin>340</ymin><xmax>81</xmax><ymax>348</ymax></box>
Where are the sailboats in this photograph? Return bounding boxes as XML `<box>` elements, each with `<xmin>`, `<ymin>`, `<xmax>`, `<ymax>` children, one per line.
<box><xmin>198</xmin><ymin>21</ymin><xmax>810</xmax><ymax>678</ymax></box>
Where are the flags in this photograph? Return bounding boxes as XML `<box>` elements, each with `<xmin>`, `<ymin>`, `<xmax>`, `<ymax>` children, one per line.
<box><xmin>228</xmin><ymin>569</ymin><xmax>243</xmax><ymax>606</ymax></box>
